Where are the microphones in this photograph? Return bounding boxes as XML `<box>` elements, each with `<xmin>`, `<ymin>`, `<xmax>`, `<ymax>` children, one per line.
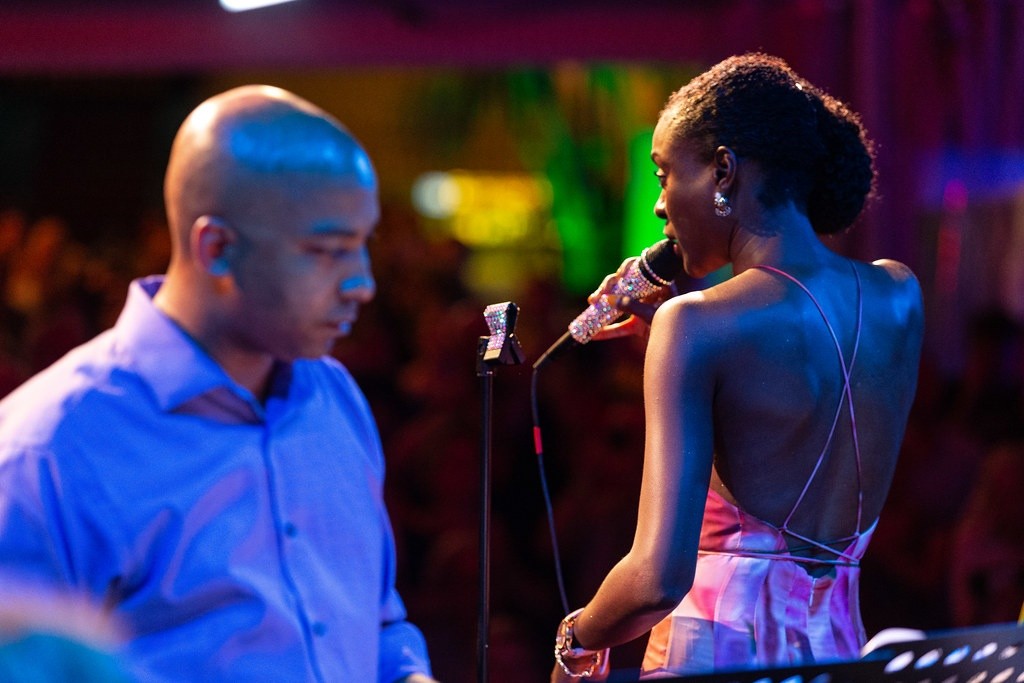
<box><xmin>533</xmin><ymin>238</ymin><xmax>684</xmax><ymax>377</ymax></box>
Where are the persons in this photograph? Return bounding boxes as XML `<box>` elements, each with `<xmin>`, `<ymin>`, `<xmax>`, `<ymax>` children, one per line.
<box><xmin>1</xmin><ymin>82</ymin><xmax>441</xmax><ymax>683</ymax></box>
<box><xmin>555</xmin><ymin>50</ymin><xmax>925</xmax><ymax>683</ymax></box>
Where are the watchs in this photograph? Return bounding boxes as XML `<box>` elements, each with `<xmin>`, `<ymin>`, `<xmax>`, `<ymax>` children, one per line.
<box><xmin>555</xmin><ymin>606</ymin><xmax>610</xmax><ymax>679</ymax></box>
